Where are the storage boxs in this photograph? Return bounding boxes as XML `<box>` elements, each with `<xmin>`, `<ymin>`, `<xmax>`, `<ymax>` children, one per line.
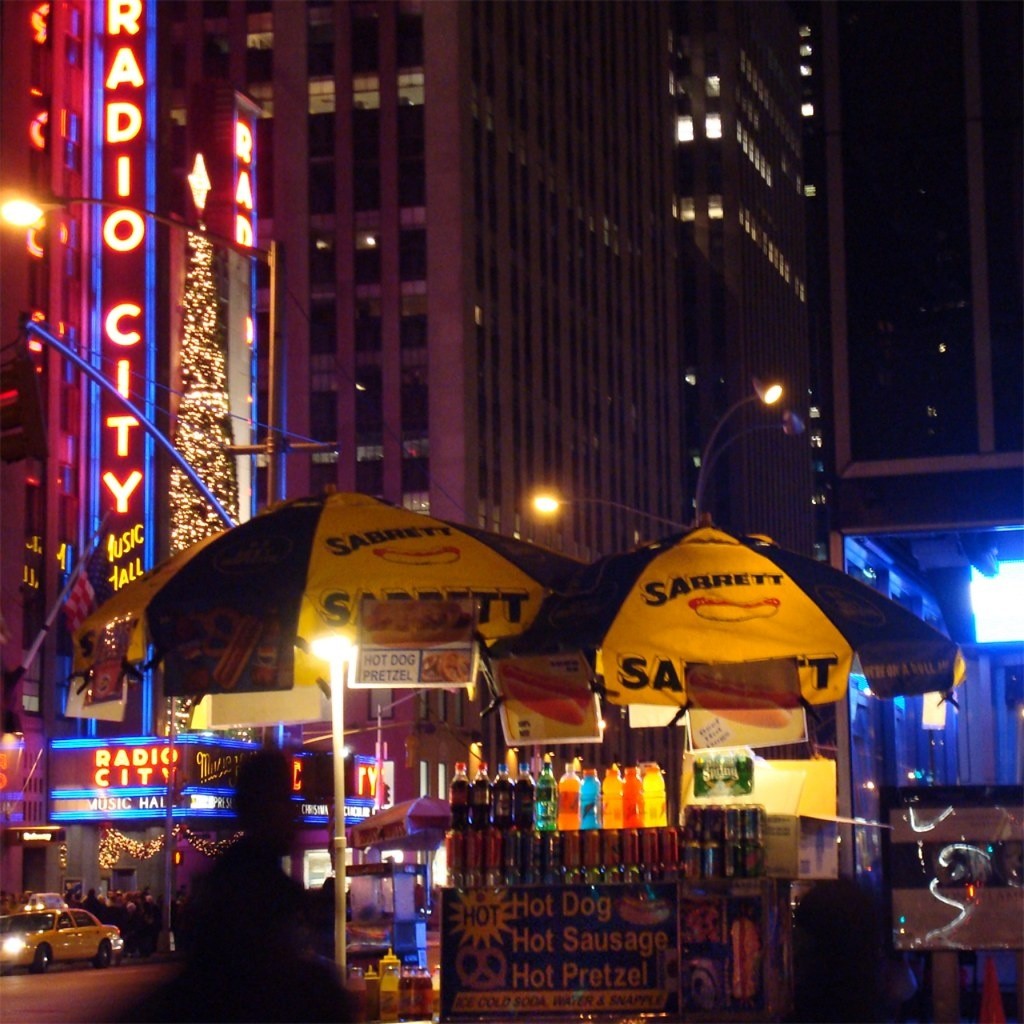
<box><xmin>686</xmin><ymin>749</ymin><xmax>897</xmax><ymax>878</ymax></box>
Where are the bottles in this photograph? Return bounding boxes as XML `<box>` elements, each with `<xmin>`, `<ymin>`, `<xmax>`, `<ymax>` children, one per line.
<box><xmin>449</xmin><ymin>762</ymin><xmax>470</xmax><ymax>828</ymax></box>
<box><xmin>602</xmin><ymin>769</ymin><xmax>623</xmax><ymax>829</ymax></box>
<box><xmin>415</xmin><ymin>965</ymin><xmax>432</xmax><ymax>1020</ymax></box>
<box><xmin>580</xmin><ymin>769</ymin><xmax>601</xmax><ymax>830</ymax></box>
<box><xmin>380</xmin><ymin>968</ymin><xmax>398</xmax><ymax>1020</ymax></box>
<box><xmin>363</xmin><ymin>965</ymin><xmax>380</xmax><ymax>1020</ymax></box>
<box><xmin>379</xmin><ymin>948</ymin><xmax>401</xmax><ymax>975</ymax></box>
<box><xmin>558</xmin><ymin>763</ymin><xmax>580</xmax><ymax>830</ymax></box>
<box><xmin>470</xmin><ymin>766</ymin><xmax>491</xmax><ymax>826</ymax></box>
<box><xmin>398</xmin><ymin>965</ymin><xmax>415</xmax><ymax>1017</ymax></box>
<box><xmin>514</xmin><ymin>763</ymin><xmax>536</xmax><ymax>828</ymax></box>
<box><xmin>643</xmin><ymin>766</ymin><xmax>668</xmax><ymax>828</ymax></box>
<box><xmin>537</xmin><ymin>753</ymin><xmax>558</xmax><ymax>830</ymax></box>
<box><xmin>731</xmin><ymin>917</ymin><xmax>759</xmax><ymax>998</ymax></box>
<box><xmin>346</xmin><ymin>967</ymin><xmax>366</xmax><ymax>1024</ymax></box>
<box><xmin>623</xmin><ymin>766</ymin><xmax>643</xmax><ymax>828</ymax></box>
<box><xmin>491</xmin><ymin>762</ymin><xmax>515</xmax><ymax>827</ymax></box>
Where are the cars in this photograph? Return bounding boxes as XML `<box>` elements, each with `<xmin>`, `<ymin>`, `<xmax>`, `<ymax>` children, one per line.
<box><xmin>0</xmin><ymin>907</ymin><xmax>123</xmax><ymax>975</ymax></box>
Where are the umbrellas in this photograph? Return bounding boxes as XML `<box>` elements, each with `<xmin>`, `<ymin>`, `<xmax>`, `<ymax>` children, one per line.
<box><xmin>485</xmin><ymin>528</ymin><xmax>964</xmax><ymax>805</ymax></box>
<box><xmin>348</xmin><ymin>798</ymin><xmax>452</xmax><ymax>909</ymax></box>
<box><xmin>74</xmin><ymin>493</ymin><xmax>588</xmax><ymax>990</ymax></box>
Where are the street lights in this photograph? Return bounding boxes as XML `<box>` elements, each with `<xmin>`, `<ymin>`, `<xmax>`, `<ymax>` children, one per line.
<box><xmin>312</xmin><ymin>632</ymin><xmax>361</xmax><ymax>971</ymax></box>
<box><xmin>1</xmin><ymin>192</ymin><xmax>285</xmax><ymax>511</ymax></box>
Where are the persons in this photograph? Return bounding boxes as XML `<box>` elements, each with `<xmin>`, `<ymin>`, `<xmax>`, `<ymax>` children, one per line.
<box><xmin>0</xmin><ymin>888</ymin><xmax>181</xmax><ymax>965</ymax></box>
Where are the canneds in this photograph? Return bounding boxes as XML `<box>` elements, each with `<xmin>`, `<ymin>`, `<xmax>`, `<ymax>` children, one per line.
<box><xmin>444</xmin><ymin>827</ymin><xmax>679</xmax><ymax>889</ymax></box>
<box><xmin>681</xmin><ymin>803</ymin><xmax>768</xmax><ymax>878</ymax></box>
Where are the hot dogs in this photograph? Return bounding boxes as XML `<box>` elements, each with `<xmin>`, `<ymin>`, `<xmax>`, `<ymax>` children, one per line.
<box><xmin>688</xmin><ymin>672</ymin><xmax>797</xmax><ymax>728</ymax></box>
<box><xmin>729</xmin><ymin>916</ymin><xmax>760</xmax><ymax>999</ymax></box>
<box><xmin>362</xmin><ymin>601</ymin><xmax>471</xmax><ymax>646</ymax></box>
<box><xmin>501</xmin><ymin>663</ymin><xmax>591</xmax><ymax>727</ymax></box>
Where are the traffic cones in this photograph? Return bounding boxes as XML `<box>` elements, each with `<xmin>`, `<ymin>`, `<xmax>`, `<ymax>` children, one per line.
<box><xmin>977</xmin><ymin>955</ymin><xmax>1005</xmax><ymax>1024</ymax></box>
